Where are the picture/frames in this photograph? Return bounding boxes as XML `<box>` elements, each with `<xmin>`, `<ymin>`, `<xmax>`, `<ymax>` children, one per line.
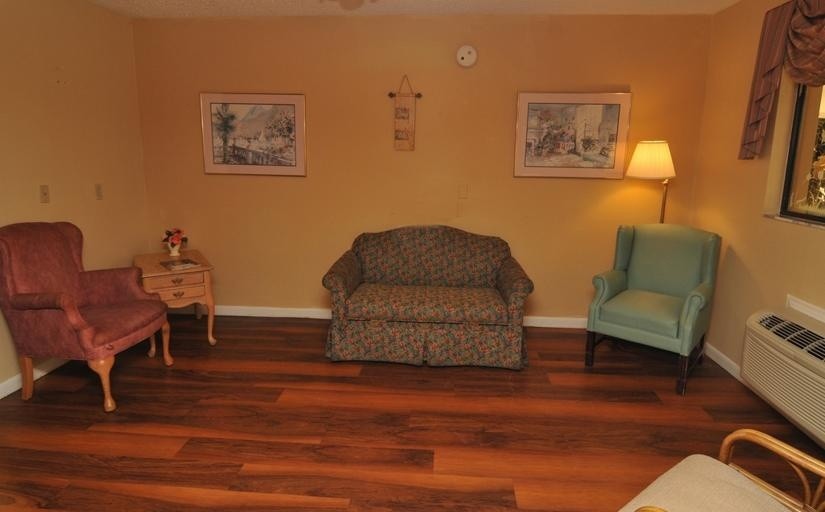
<box><xmin>512</xmin><ymin>87</ymin><xmax>635</xmax><ymax>180</ymax></box>
<box><xmin>198</xmin><ymin>88</ymin><xmax>307</xmax><ymax>177</ymax></box>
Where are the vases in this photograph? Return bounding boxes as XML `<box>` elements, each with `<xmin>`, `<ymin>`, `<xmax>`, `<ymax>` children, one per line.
<box><xmin>166</xmin><ymin>238</ymin><xmax>182</xmax><ymax>257</ymax></box>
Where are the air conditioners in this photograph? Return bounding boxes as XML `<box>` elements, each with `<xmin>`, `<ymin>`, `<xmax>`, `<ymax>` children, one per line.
<box><xmin>738</xmin><ymin>307</ymin><xmax>825</xmax><ymax>453</ymax></box>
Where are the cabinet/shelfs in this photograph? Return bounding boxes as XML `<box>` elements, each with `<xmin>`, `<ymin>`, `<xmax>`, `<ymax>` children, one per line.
<box><xmin>131</xmin><ymin>251</ymin><xmax>220</xmax><ymax>359</ymax></box>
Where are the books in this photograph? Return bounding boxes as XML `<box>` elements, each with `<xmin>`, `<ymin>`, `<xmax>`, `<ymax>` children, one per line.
<box><xmin>160</xmin><ymin>258</ymin><xmax>200</xmax><ymax>271</ymax></box>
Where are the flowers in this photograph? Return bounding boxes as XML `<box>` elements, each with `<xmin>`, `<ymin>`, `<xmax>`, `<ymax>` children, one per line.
<box><xmin>159</xmin><ymin>228</ymin><xmax>190</xmax><ymax>249</ymax></box>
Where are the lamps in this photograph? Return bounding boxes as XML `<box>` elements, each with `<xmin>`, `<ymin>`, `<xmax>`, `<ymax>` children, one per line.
<box><xmin>623</xmin><ymin>138</ymin><xmax>679</xmax><ymax>227</ymax></box>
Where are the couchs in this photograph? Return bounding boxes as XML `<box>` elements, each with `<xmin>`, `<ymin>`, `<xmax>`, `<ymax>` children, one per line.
<box><xmin>319</xmin><ymin>223</ymin><xmax>535</xmax><ymax>375</ymax></box>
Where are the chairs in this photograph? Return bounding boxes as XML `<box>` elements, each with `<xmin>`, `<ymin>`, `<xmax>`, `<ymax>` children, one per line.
<box><xmin>1</xmin><ymin>220</ymin><xmax>176</xmax><ymax>416</ymax></box>
<box><xmin>580</xmin><ymin>222</ymin><xmax>721</xmax><ymax>396</ymax></box>
<box><xmin>607</xmin><ymin>427</ymin><xmax>825</xmax><ymax>512</ymax></box>
